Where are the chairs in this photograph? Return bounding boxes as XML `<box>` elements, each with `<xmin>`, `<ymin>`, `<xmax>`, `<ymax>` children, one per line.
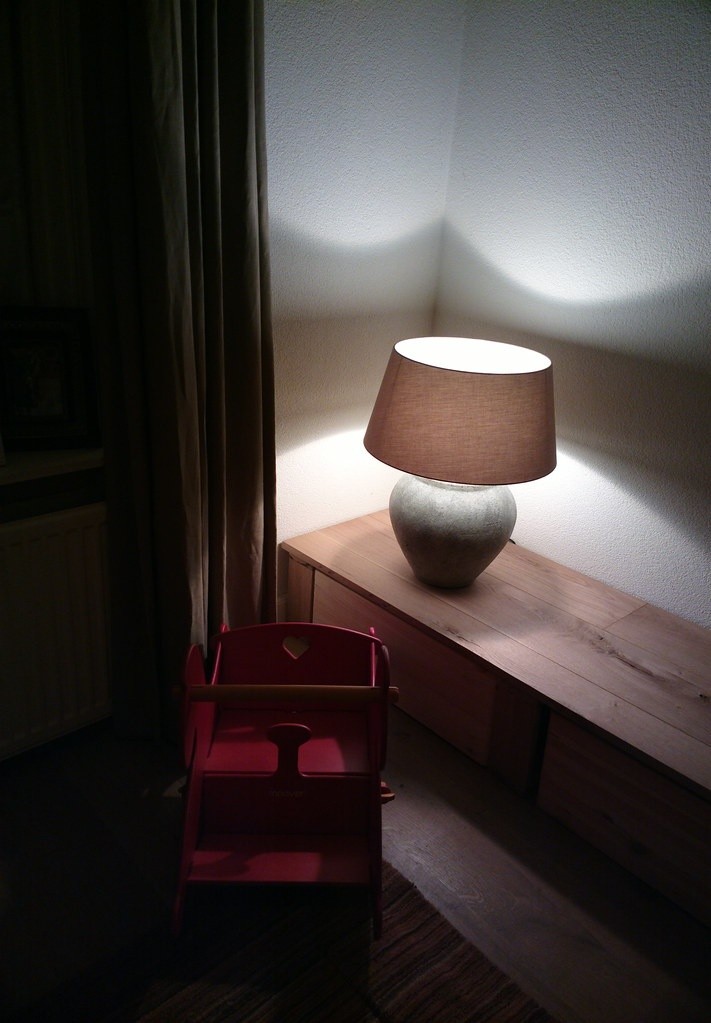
<box><xmin>172</xmin><ymin>620</ymin><xmax>398</xmax><ymax>942</ymax></box>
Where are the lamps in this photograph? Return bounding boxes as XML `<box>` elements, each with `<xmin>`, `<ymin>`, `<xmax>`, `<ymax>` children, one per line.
<box><xmin>364</xmin><ymin>336</ymin><xmax>558</xmax><ymax>594</ymax></box>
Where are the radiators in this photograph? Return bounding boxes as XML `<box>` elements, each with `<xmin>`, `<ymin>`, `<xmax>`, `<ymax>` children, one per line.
<box><xmin>0</xmin><ymin>502</ymin><xmax>113</xmax><ymax>761</ymax></box>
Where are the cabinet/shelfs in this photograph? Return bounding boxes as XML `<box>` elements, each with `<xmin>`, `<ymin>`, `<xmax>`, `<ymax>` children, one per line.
<box><xmin>280</xmin><ymin>507</ymin><xmax>711</xmax><ymax>930</ymax></box>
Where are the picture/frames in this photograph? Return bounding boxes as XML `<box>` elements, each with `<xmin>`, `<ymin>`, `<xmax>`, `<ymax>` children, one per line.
<box><xmin>0</xmin><ymin>305</ymin><xmax>101</xmax><ymax>451</ymax></box>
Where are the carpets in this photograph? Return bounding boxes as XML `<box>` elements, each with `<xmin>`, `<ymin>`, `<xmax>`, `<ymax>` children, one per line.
<box><xmin>0</xmin><ymin>858</ymin><xmax>565</xmax><ymax>1023</ymax></box>
